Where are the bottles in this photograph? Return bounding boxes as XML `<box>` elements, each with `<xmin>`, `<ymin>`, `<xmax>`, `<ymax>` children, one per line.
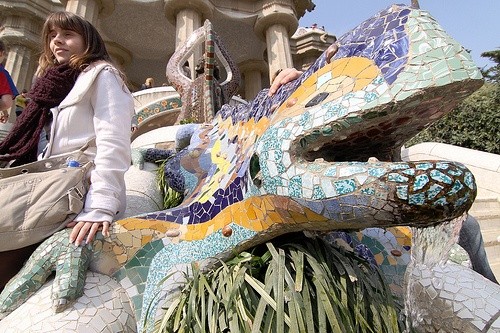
<box><xmin>68</xmin><ymin>161</ymin><xmax>79</xmax><ymax>168</ymax></box>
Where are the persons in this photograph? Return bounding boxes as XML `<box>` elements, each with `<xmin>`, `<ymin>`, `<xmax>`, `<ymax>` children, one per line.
<box><xmin>0</xmin><ymin>39</ymin><xmax>19</xmax><ymax>122</ymax></box>
<box><xmin>265</xmin><ymin>68</ymin><xmax>498</xmax><ymax>290</ymax></box>
<box><xmin>0</xmin><ymin>12</ymin><xmax>134</xmax><ymax>290</ymax></box>
<box><xmin>142</xmin><ymin>78</ymin><xmax>154</xmax><ymax>91</ymax></box>
<box><xmin>14</xmin><ymin>88</ymin><xmax>30</xmax><ymax>120</ymax></box>
